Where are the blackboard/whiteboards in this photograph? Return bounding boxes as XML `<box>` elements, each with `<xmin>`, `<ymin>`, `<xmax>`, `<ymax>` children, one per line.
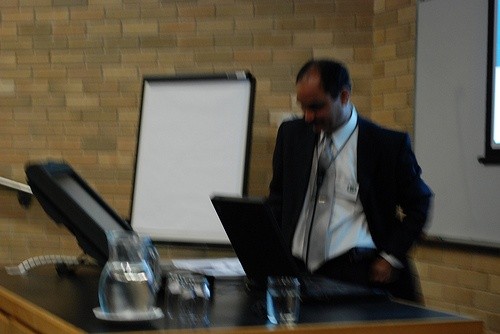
<box><xmin>129</xmin><ymin>72</ymin><xmax>256</xmax><ymax>253</ymax></box>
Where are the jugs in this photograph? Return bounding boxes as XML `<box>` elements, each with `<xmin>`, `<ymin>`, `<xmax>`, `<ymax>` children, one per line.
<box><xmin>97</xmin><ymin>230</ymin><xmax>155</xmax><ymax>315</ymax></box>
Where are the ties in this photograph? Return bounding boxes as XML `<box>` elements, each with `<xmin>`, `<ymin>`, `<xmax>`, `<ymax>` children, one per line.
<box><xmin>302</xmin><ymin>137</ymin><xmax>335</xmax><ymax>273</ymax></box>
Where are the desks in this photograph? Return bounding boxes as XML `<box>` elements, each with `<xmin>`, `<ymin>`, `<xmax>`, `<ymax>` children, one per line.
<box><xmin>0</xmin><ymin>238</ymin><xmax>484</xmax><ymax>334</ymax></box>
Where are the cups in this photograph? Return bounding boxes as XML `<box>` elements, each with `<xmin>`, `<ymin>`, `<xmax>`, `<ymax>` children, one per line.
<box><xmin>266</xmin><ymin>277</ymin><xmax>300</xmax><ymax>325</ymax></box>
<box><xmin>186</xmin><ymin>276</ymin><xmax>211</xmax><ymax>306</ymax></box>
<box><xmin>166</xmin><ymin>271</ymin><xmax>194</xmax><ymax>298</ymax></box>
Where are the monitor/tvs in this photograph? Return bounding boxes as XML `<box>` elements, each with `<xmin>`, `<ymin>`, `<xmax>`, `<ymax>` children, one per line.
<box><xmin>26</xmin><ymin>163</ymin><xmax>138</xmax><ymax>266</ymax></box>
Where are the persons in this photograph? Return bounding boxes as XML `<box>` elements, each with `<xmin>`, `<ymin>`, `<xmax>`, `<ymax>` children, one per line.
<box><xmin>264</xmin><ymin>59</ymin><xmax>432</xmax><ymax>302</ymax></box>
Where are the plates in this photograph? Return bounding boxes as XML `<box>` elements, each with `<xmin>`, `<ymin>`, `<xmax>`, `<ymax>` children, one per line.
<box><xmin>92</xmin><ymin>306</ymin><xmax>162</xmax><ymax>320</ymax></box>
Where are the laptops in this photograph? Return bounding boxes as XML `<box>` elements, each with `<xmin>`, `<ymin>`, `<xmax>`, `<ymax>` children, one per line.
<box><xmin>212</xmin><ymin>196</ymin><xmax>384</xmax><ymax>307</ymax></box>
